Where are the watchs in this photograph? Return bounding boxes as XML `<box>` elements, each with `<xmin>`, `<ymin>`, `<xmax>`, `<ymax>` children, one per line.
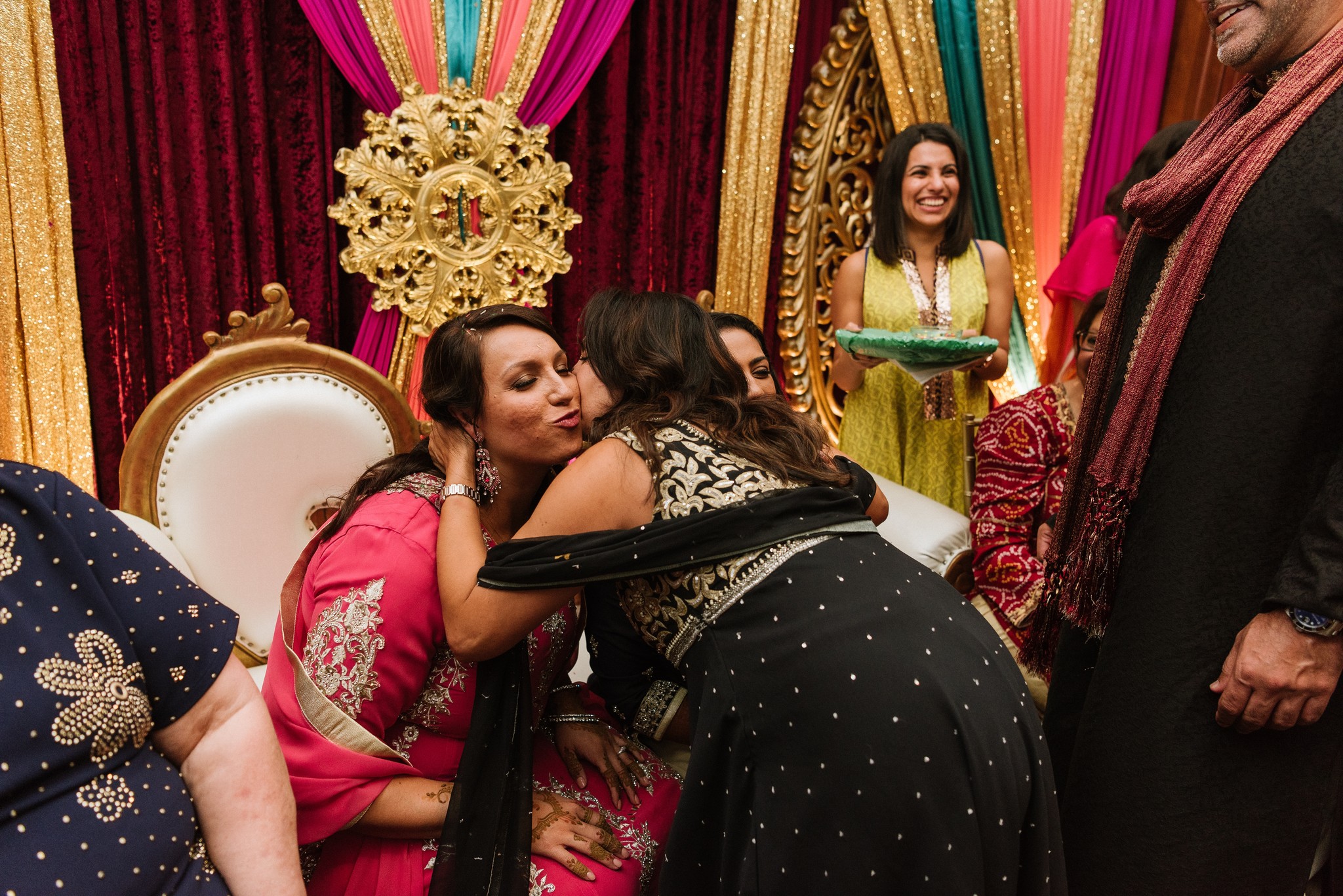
<box><xmin>974</xmin><ymin>353</ymin><xmax>994</xmax><ymax>369</ymax></box>
<box><xmin>1282</xmin><ymin>604</ymin><xmax>1343</xmax><ymax>639</ymax></box>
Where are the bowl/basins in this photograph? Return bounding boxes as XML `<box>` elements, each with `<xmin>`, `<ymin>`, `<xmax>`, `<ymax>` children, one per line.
<box><xmin>910</xmin><ymin>326</ymin><xmax>963</xmax><ymax>341</ymax></box>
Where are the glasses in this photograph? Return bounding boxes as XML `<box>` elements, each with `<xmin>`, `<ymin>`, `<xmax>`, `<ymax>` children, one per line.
<box><xmin>1075</xmin><ymin>330</ymin><xmax>1098</xmax><ymax>352</ymax></box>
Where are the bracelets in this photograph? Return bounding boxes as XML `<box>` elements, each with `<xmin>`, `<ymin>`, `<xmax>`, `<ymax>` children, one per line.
<box><xmin>549</xmin><ymin>714</ymin><xmax>602</xmax><ymax>722</ymax></box>
<box><xmin>439</xmin><ymin>484</ymin><xmax>481</xmax><ymax>508</ymax></box>
<box><xmin>549</xmin><ymin>684</ymin><xmax>582</xmax><ymax>694</ymax></box>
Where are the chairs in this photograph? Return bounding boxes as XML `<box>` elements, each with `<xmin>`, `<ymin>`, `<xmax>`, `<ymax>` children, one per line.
<box><xmin>119</xmin><ymin>282</ymin><xmax>430</xmax><ymax>689</ymax></box>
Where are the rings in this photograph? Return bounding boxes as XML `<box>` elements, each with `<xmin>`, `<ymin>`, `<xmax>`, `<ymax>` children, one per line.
<box><xmin>617</xmin><ymin>746</ymin><xmax>627</xmax><ymax>756</ymax></box>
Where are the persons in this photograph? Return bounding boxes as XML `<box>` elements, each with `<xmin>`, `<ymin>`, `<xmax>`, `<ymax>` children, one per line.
<box><xmin>971</xmin><ymin>285</ymin><xmax>1113</xmax><ymax>699</ymax></box>
<box><xmin>831</xmin><ymin>122</ymin><xmax>1014</xmax><ymax>518</ymax></box>
<box><xmin>1043</xmin><ymin>119</ymin><xmax>1204</xmax><ymax>380</ymax></box>
<box><xmin>1025</xmin><ymin>0</ymin><xmax>1343</xmax><ymax>896</ymax></box>
<box><xmin>261</xmin><ymin>304</ymin><xmax>685</xmax><ymax>896</ymax></box>
<box><xmin>0</xmin><ymin>457</ymin><xmax>308</xmax><ymax>896</ymax></box>
<box><xmin>584</xmin><ymin>312</ymin><xmax>785</xmax><ymax>778</ymax></box>
<box><xmin>429</xmin><ymin>288</ymin><xmax>1067</xmax><ymax>896</ymax></box>
